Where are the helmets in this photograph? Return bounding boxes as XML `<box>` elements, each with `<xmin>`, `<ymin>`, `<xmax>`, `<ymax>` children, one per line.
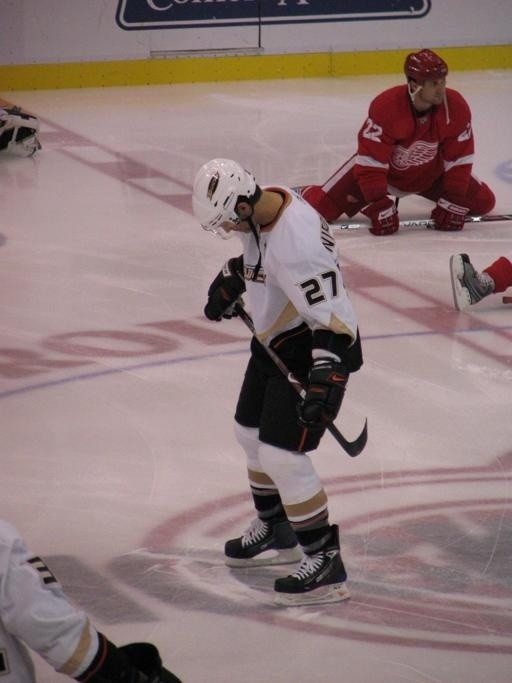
<box><xmin>404</xmin><ymin>48</ymin><xmax>448</xmax><ymax>87</ymax></box>
<box><xmin>191</xmin><ymin>157</ymin><xmax>257</xmax><ymax>240</ymax></box>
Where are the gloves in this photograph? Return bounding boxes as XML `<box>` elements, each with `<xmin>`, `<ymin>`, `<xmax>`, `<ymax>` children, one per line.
<box><xmin>360</xmin><ymin>195</ymin><xmax>399</xmax><ymax>236</ymax></box>
<box><xmin>76</xmin><ymin>630</ymin><xmax>162</xmax><ymax>683</ymax></box>
<box><xmin>203</xmin><ymin>254</ymin><xmax>247</xmax><ymax>322</ymax></box>
<box><xmin>427</xmin><ymin>199</ymin><xmax>470</xmax><ymax>232</ymax></box>
<box><xmin>295</xmin><ymin>356</ymin><xmax>349</xmax><ymax>429</ymax></box>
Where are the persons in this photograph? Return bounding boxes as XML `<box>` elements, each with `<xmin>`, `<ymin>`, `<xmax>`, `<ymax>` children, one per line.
<box><xmin>462</xmin><ymin>251</ymin><xmax>512</xmax><ymax>307</ymax></box>
<box><xmin>0</xmin><ymin>100</ymin><xmax>41</xmax><ymax>162</ymax></box>
<box><xmin>188</xmin><ymin>159</ymin><xmax>362</xmax><ymax>592</ymax></box>
<box><xmin>292</xmin><ymin>51</ymin><xmax>496</xmax><ymax>232</ymax></box>
<box><xmin>1</xmin><ymin>521</ymin><xmax>178</xmax><ymax>682</ymax></box>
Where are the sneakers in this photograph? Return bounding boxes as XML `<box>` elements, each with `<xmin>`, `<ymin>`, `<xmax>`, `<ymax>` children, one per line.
<box><xmin>0</xmin><ymin>108</ymin><xmax>40</xmax><ymax>151</ymax></box>
<box><xmin>456</xmin><ymin>254</ymin><xmax>494</xmax><ymax>306</ymax></box>
<box><xmin>224</xmin><ymin>494</ymin><xmax>297</xmax><ymax>559</ymax></box>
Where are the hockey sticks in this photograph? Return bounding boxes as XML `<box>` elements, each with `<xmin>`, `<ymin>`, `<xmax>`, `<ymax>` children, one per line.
<box><xmin>235</xmin><ymin>302</ymin><xmax>369</xmax><ymax>458</ymax></box>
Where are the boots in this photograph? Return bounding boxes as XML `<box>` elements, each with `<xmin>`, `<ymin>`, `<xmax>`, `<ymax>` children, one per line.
<box><xmin>273</xmin><ymin>523</ymin><xmax>347</xmax><ymax>593</ymax></box>
